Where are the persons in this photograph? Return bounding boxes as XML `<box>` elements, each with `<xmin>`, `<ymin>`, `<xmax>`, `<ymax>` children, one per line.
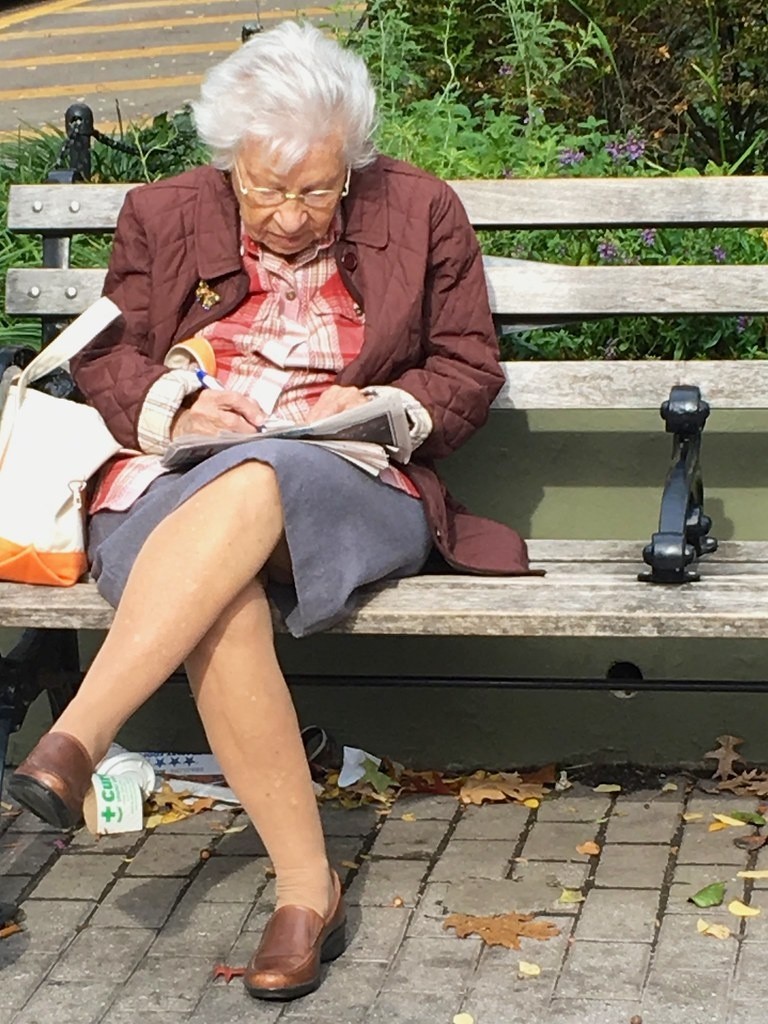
<box><xmin>8</xmin><ymin>17</ymin><xmax>547</xmax><ymax>1002</ymax></box>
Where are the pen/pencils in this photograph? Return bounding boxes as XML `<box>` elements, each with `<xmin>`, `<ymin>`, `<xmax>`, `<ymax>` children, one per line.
<box><xmin>196</xmin><ymin>364</ymin><xmax>265</xmax><ymax>435</ymax></box>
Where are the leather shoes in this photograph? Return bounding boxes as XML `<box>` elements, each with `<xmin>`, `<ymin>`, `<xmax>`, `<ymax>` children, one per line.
<box><xmin>245</xmin><ymin>869</ymin><xmax>344</xmax><ymax>998</ymax></box>
<box><xmin>7</xmin><ymin>732</ymin><xmax>94</xmax><ymax>828</ymax></box>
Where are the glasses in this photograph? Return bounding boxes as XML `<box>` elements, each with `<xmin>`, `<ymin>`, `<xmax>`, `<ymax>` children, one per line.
<box><xmin>231</xmin><ymin>158</ymin><xmax>352</xmax><ymax>210</ymax></box>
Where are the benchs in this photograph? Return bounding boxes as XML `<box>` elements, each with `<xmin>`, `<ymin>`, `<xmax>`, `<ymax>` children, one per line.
<box><xmin>0</xmin><ymin>171</ymin><xmax>768</xmax><ymax>774</ymax></box>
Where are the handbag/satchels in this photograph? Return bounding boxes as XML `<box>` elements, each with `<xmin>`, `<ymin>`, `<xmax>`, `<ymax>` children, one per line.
<box><xmin>0</xmin><ymin>295</ymin><xmax>122</xmax><ymax>584</ymax></box>
